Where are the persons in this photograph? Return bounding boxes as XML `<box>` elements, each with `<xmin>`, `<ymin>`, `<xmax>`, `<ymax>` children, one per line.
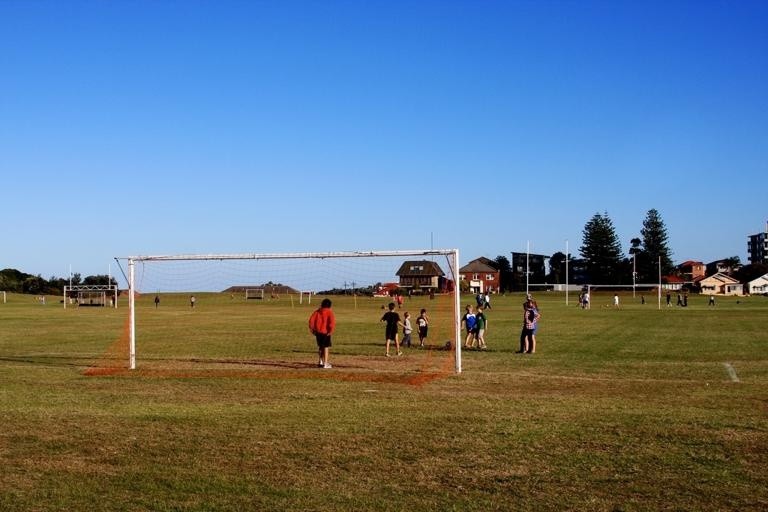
<box><xmin>525</xmin><ymin>293</ymin><xmax>532</xmax><ymax>300</ymax></box>
<box><xmin>471</xmin><ymin>306</ymin><xmax>489</xmax><ymax>349</ymax></box>
<box><xmin>576</xmin><ymin>294</ymin><xmax>582</xmax><ymax>307</ymax></box>
<box><xmin>514</xmin><ymin>301</ymin><xmax>535</xmax><ymax>354</ymax></box>
<box><xmin>416</xmin><ymin>309</ymin><xmax>429</xmax><ymax>346</ymax></box>
<box><xmin>190</xmin><ymin>295</ymin><xmax>195</xmax><ymax>306</ymax></box>
<box><xmin>524</xmin><ymin>300</ymin><xmax>540</xmax><ymax>353</ymax></box>
<box><xmin>665</xmin><ymin>288</ymin><xmax>675</xmax><ymax>308</ymax></box>
<box><xmin>613</xmin><ymin>294</ymin><xmax>619</xmax><ymax>309</ymax></box>
<box><xmin>580</xmin><ymin>292</ymin><xmax>585</xmax><ymax>299</ymax></box>
<box><xmin>381</xmin><ymin>303</ymin><xmax>413</xmax><ymax>358</ymax></box>
<box><xmin>641</xmin><ymin>294</ymin><xmax>645</xmax><ymax>304</ymax></box>
<box><xmin>677</xmin><ymin>293</ymin><xmax>683</xmax><ymax>307</ymax></box>
<box><xmin>483</xmin><ymin>291</ymin><xmax>491</xmax><ymax>310</ymax></box>
<box><xmin>396</xmin><ymin>293</ymin><xmax>403</xmax><ymax>308</ymax></box>
<box><xmin>475</xmin><ymin>291</ymin><xmax>483</xmax><ymax>309</ymax></box>
<box><xmin>684</xmin><ymin>292</ymin><xmax>688</xmax><ymax>306</ymax></box>
<box><xmin>308</xmin><ymin>298</ymin><xmax>335</xmax><ymax>369</ymax></box>
<box><xmin>583</xmin><ymin>291</ymin><xmax>590</xmax><ymax>308</ymax></box>
<box><xmin>461</xmin><ymin>304</ymin><xmax>477</xmax><ymax>349</ymax></box>
<box><xmin>401</xmin><ymin>312</ymin><xmax>413</xmax><ymax>347</ymax></box>
<box><xmin>708</xmin><ymin>295</ymin><xmax>716</xmax><ymax>306</ymax></box>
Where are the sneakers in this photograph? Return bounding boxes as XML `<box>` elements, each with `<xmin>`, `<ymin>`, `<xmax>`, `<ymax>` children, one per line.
<box><xmin>319</xmin><ymin>360</ymin><xmax>333</xmax><ymax>369</ymax></box>
<box><xmin>385</xmin><ymin>351</ymin><xmax>391</xmax><ymax>357</ymax></box>
<box><xmin>420</xmin><ymin>344</ymin><xmax>424</xmax><ymax>347</ymax></box>
<box><xmin>396</xmin><ymin>351</ymin><xmax>403</xmax><ymax>356</ymax></box>
